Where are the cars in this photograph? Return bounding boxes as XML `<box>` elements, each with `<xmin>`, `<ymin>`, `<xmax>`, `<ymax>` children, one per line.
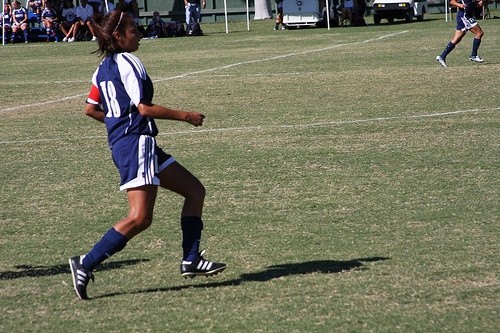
<box><xmin>371</xmin><ymin>0</ymin><xmax>428</xmax><ymax>25</ymax></box>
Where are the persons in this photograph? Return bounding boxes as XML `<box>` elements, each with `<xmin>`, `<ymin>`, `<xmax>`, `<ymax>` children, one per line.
<box><xmin>273</xmin><ymin>0</ymin><xmax>286</xmax><ymax>29</ymax></box>
<box><xmin>148</xmin><ymin>11</ymin><xmax>166</xmax><ymax>38</ymax></box>
<box><xmin>70</xmin><ymin>0</ymin><xmax>97</xmax><ymax>42</ymax></box>
<box><xmin>26</xmin><ymin>0</ymin><xmax>44</xmax><ymax>29</ymax></box>
<box><xmin>52</xmin><ymin>0</ymin><xmax>76</xmax><ymax>43</ymax></box>
<box><xmin>184</xmin><ymin>0</ymin><xmax>206</xmax><ymax>36</ymax></box>
<box><xmin>436</xmin><ymin>0</ymin><xmax>498</xmax><ymax>64</ymax></box>
<box><xmin>67</xmin><ymin>12</ymin><xmax>227</xmax><ymax>301</ymax></box>
<box><xmin>42</xmin><ymin>0</ymin><xmax>60</xmax><ymax>42</ymax></box>
<box><xmin>0</xmin><ymin>3</ymin><xmax>12</xmax><ymax>42</ymax></box>
<box><xmin>11</xmin><ymin>0</ymin><xmax>29</xmax><ymax>44</ymax></box>
<box><xmin>87</xmin><ymin>0</ymin><xmax>116</xmax><ymax>19</ymax></box>
<box><xmin>116</xmin><ymin>0</ymin><xmax>139</xmax><ymax>28</ymax></box>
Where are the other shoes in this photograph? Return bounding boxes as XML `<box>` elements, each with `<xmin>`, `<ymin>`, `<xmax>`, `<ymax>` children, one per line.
<box><xmin>92</xmin><ymin>36</ymin><xmax>97</xmax><ymax>42</ymax></box>
<box><xmin>274</xmin><ymin>27</ymin><xmax>278</xmax><ymax>31</ymax></box>
<box><xmin>282</xmin><ymin>25</ymin><xmax>286</xmax><ymax>30</ymax></box>
<box><xmin>70</xmin><ymin>37</ymin><xmax>75</xmax><ymax>42</ymax></box>
<box><xmin>68</xmin><ymin>38</ymin><xmax>71</xmax><ymax>43</ymax></box>
<box><xmin>47</xmin><ymin>39</ymin><xmax>49</xmax><ymax>43</ymax></box>
<box><xmin>63</xmin><ymin>37</ymin><xmax>68</xmax><ymax>42</ymax></box>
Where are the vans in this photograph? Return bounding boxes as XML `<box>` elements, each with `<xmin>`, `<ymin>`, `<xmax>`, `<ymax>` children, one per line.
<box><xmin>282</xmin><ymin>0</ymin><xmax>340</xmax><ymax>31</ymax></box>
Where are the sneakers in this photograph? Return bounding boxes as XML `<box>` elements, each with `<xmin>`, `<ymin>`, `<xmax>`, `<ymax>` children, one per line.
<box><xmin>436</xmin><ymin>56</ymin><xmax>448</xmax><ymax>67</ymax></box>
<box><xmin>469</xmin><ymin>55</ymin><xmax>484</xmax><ymax>63</ymax></box>
<box><xmin>68</xmin><ymin>255</ymin><xmax>95</xmax><ymax>301</ymax></box>
<box><xmin>180</xmin><ymin>250</ymin><xmax>226</xmax><ymax>278</ymax></box>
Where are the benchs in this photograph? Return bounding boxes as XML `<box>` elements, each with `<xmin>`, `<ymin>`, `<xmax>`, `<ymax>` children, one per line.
<box><xmin>0</xmin><ymin>11</ymin><xmax>54</xmax><ymax>42</ymax></box>
